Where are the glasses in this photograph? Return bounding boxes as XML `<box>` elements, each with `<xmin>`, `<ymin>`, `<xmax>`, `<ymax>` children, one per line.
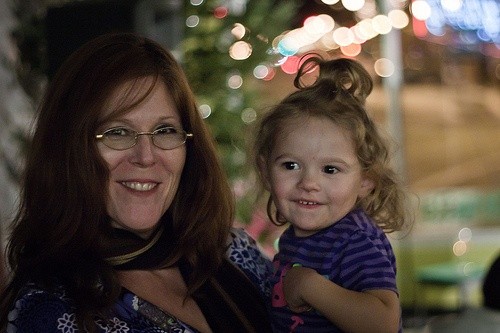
<box><xmin>94</xmin><ymin>127</ymin><xmax>194</xmax><ymax>150</ymax></box>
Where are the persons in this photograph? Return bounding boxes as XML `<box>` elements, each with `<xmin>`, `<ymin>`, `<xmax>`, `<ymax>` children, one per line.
<box><xmin>249</xmin><ymin>53</ymin><xmax>421</xmax><ymax>333</ymax></box>
<box><xmin>1</xmin><ymin>33</ymin><xmax>273</xmax><ymax>333</ymax></box>
